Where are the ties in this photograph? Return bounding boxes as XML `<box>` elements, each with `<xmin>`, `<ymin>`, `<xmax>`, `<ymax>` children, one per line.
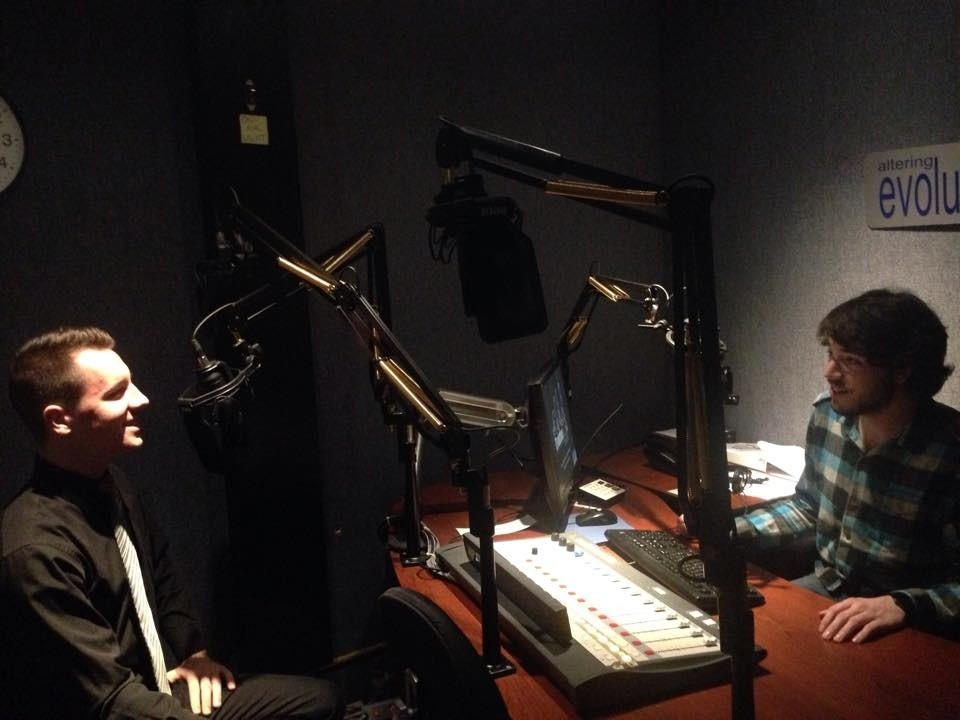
<box><xmin>103</xmin><ymin>481</ymin><xmax>173</xmax><ymax>696</ymax></box>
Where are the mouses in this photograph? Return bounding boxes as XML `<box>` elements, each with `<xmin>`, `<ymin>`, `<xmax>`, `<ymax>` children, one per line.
<box><xmin>576</xmin><ymin>510</ymin><xmax>617</xmax><ymax>526</ymax></box>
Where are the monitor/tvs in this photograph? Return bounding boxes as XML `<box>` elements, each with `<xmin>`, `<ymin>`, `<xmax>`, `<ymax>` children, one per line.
<box><xmin>526</xmin><ymin>356</ymin><xmax>581</xmax><ymax>534</ymax></box>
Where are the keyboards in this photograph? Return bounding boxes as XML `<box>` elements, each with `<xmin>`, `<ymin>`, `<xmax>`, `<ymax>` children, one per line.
<box><xmin>604</xmin><ymin>529</ymin><xmax>766</xmax><ymax>610</ymax></box>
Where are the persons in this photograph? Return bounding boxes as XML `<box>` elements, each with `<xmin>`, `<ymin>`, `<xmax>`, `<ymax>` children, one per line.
<box><xmin>0</xmin><ymin>327</ymin><xmax>356</xmax><ymax>719</ymax></box>
<box><xmin>677</xmin><ymin>287</ymin><xmax>958</xmax><ymax>643</ymax></box>
